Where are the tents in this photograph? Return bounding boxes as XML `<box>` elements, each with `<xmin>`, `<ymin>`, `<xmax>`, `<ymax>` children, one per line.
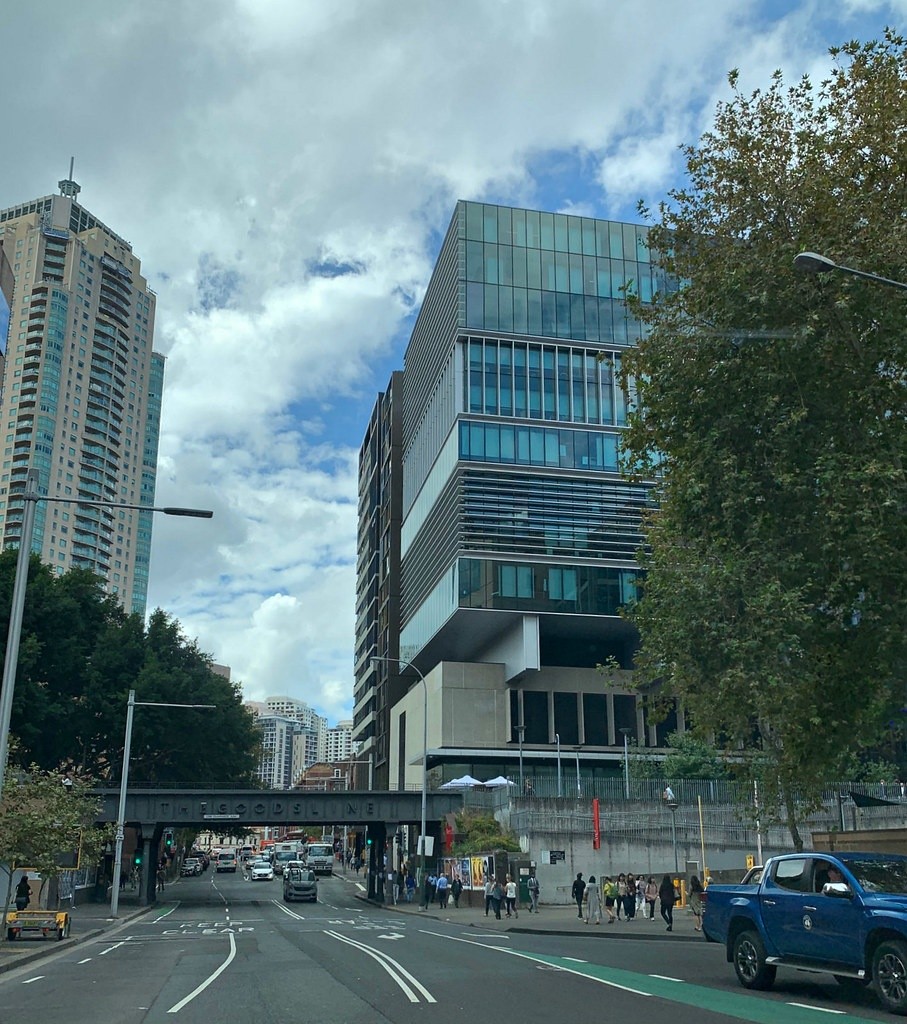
<box><xmin>438</xmin><ymin>776</ymin><xmax>517</xmax><ymax>792</ymax></box>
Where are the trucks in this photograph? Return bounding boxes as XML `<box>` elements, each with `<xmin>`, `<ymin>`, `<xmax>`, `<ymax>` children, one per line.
<box><xmin>259</xmin><ymin>840</ymin><xmax>334</xmax><ymax>876</ymax></box>
<box><xmin>241</xmin><ymin>844</ymin><xmax>257</xmax><ymax>859</ymax></box>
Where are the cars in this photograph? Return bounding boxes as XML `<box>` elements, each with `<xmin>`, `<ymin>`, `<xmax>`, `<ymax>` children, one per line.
<box><xmin>245</xmin><ymin>855</ymin><xmax>275</xmax><ymax>881</ymax></box>
<box><xmin>192</xmin><ymin>847</ymin><xmax>221</xmax><ymax>870</ymax></box>
<box><xmin>283</xmin><ymin>861</ymin><xmax>306</xmax><ymax>881</ymax></box>
<box><xmin>182</xmin><ymin>858</ymin><xmax>204</xmax><ymax>874</ymax></box>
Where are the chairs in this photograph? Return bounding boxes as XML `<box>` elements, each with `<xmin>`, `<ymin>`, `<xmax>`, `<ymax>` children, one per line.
<box><xmin>814</xmin><ymin>869</ymin><xmax>830</xmax><ymax>892</ymax></box>
<box><xmin>866</xmin><ymin>866</ymin><xmax>899</xmax><ymax>887</ymax></box>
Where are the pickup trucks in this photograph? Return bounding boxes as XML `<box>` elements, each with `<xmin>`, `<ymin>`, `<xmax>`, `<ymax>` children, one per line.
<box><xmin>699</xmin><ymin>852</ymin><xmax>907</xmax><ymax>1016</ymax></box>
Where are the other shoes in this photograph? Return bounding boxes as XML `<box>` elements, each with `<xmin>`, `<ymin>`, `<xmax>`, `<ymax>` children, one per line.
<box><xmin>649</xmin><ymin>917</ymin><xmax>654</xmax><ymax>921</ymax></box>
<box><xmin>644</xmin><ymin>915</ymin><xmax>648</xmax><ymax>919</ymax></box>
<box><xmin>515</xmin><ymin>912</ymin><xmax>518</xmax><ymax>919</ymax></box>
<box><xmin>504</xmin><ymin>912</ymin><xmax>510</xmax><ymax>916</ymax></box>
<box><xmin>595</xmin><ymin>920</ymin><xmax>600</xmax><ymax>925</ymax></box>
<box><xmin>534</xmin><ymin>911</ymin><xmax>539</xmax><ymax>913</ymax></box>
<box><xmin>528</xmin><ymin>908</ymin><xmax>532</xmax><ymax>912</ymax></box>
<box><xmin>576</xmin><ymin>915</ymin><xmax>583</xmax><ymax>920</ymax></box>
<box><xmin>666</xmin><ymin>925</ymin><xmax>672</xmax><ymax>931</ymax></box>
<box><xmin>439</xmin><ymin>903</ymin><xmax>447</xmax><ymax>910</ymax></box>
<box><xmin>616</xmin><ymin>917</ymin><xmax>636</xmax><ymax>922</ymax></box>
<box><xmin>583</xmin><ymin>920</ymin><xmax>589</xmax><ymax>925</ymax></box>
<box><xmin>608</xmin><ymin>916</ymin><xmax>615</xmax><ymax>923</ymax></box>
<box><xmin>694</xmin><ymin>927</ymin><xmax>701</xmax><ymax>932</ymax></box>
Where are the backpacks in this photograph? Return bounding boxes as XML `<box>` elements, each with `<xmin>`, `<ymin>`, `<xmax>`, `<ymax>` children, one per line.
<box><xmin>607</xmin><ymin>883</ymin><xmax>618</xmax><ymax>900</ymax></box>
<box><xmin>493</xmin><ymin>884</ymin><xmax>505</xmax><ymax>900</ymax></box>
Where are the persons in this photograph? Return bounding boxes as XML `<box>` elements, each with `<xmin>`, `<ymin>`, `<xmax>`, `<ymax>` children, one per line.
<box><xmin>14</xmin><ymin>876</ymin><xmax>33</xmax><ymax>911</ymax></box>
<box><xmin>483</xmin><ymin>873</ymin><xmax>519</xmax><ymax>920</ymax></box>
<box><xmin>120</xmin><ymin>865</ymin><xmax>140</xmax><ymax>891</ymax></box>
<box><xmin>527</xmin><ymin>872</ymin><xmax>540</xmax><ymax>913</ymax></box>
<box><xmin>821</xmin><ymin>862</ymin><xmax>849</xmax><ymax>893</ymax></box>
<box><xmin>333</xmin><ymin>841</ymin><xmax>360</xmax><ymax>876</ymax></box>
<box><xmin>158</xmin><ymin>868</ymin><xmax>165</xmax><ymax>891</ymax></box>
<box><xmin>483</xmin><ymin>859</ymin><xmax>488</xmax><ymax>881</ymax></box>
<box><xmin>572</xmin><ymin>872</ymin><xmax>681</xmax><ymax>931</ymax></box>
<box><xmin>375</xmin><ymin>862</ymin><xmax>463</xmax><ymax>909</ymax></box>
<box><xmin>684</xmin><ymin>875</ymin><xmax>714</xmax><ymax>932</ymax></box>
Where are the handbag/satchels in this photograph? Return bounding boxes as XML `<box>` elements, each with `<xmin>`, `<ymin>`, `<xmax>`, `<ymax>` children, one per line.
<box><xmin>534</xmin><ymin>890</ymin><xmax>539</xmax><ymax>894</ymax></box>
<box><xmin>447</xmin><ymin>895</ymin><xmax>454</xmax><ymax>905</ymax></box>
<box><xmin>673</xmin><ymin>888</ymin><xmax>681</xmax><ymax>901</ymax></box>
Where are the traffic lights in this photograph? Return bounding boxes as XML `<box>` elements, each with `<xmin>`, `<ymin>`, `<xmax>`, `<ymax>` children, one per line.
<box><xmin>134</xmin><ymin>848</ymin><xmax>143</xmax><ymax>865</ymax></box>
<box><xmin>396</xmin><ymin>830</ymin><xmax>402</xmax><ymax>846</ymax></box>
<box><xmin>165</xmin><ymin>833</ymin><xmax>173</xmax><ymax>846</ymax></box>
<box><xmin>366</xmin><ymin>831</ymin><xmax>373</xmax><ymax>845</ymax></box>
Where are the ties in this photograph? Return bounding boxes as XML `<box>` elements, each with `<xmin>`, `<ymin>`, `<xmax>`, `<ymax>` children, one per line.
<box><xmin>533</xmin><ymin>878</ymin><xmax>535</xmax><ymax>884</ymax></box>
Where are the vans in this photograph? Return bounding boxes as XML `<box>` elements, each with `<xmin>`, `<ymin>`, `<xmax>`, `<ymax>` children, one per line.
<box><xmin>217</xmin><ymin>850</ymin><xmax>238</xmax><ymax>872</ymax></box>
<box><xmin>283</xmin><ymin>868</ymin><xmax>317</xmax><ymax>903</ymax></box>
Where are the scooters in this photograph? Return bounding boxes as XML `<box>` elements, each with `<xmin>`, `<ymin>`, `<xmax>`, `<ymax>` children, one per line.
<box><xmin>180</xmin><ymin>865</ymin><xmax>199</xmax><ymax>877</ymax></box>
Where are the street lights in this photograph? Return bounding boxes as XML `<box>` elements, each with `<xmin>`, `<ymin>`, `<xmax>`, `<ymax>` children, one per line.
<box><xmin>0</xmin><ymin>467</ymin><xmax>214</xmax><ymax>789</ymax></box>
<box><xmin>109</xmin><ymin>689</ymin><xmax>217</xmax><ymax>919</ymax></box>
<box><xmin>514</xmin><ymin>726</ymin><xmax>526</xmax><ymax>795</ymax></box>
<box><xmin>370</xmin><ymin>656</ymin><xmax>427</xmax><ymax>912</ymax></box>
<box><xmin>572</xmin><ymin>728</ymin><xmax>632</xmax><ymax>799</ymax></box>
<box><xmin>667</xmin><ymin>804</ymin><xmax>680</xmax><ymax>879</ymax></box>
